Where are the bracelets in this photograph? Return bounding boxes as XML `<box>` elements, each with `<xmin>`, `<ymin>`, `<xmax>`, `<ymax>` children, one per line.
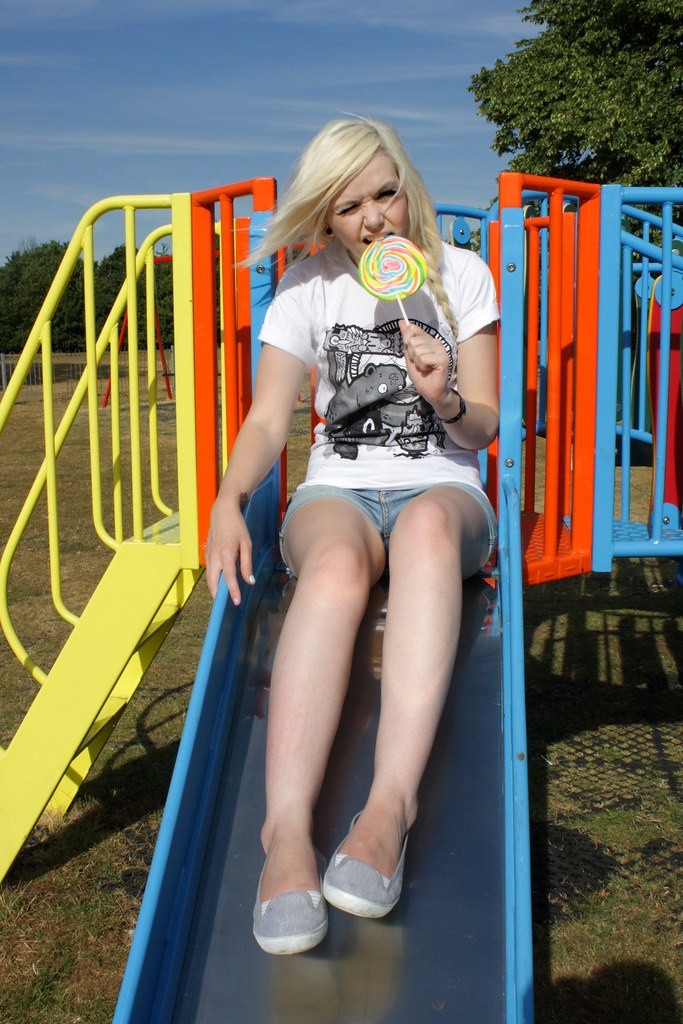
<box><xmin>438</xmin><ymin>386</ymin><xmax>466</xmax><ymax>425</ymax></box>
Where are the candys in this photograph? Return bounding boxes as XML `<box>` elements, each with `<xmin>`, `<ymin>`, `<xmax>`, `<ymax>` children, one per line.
<box><xmin>358</xmin><ymin>236</ymin><xmax>427</xmax><ymax>301</ymax></box>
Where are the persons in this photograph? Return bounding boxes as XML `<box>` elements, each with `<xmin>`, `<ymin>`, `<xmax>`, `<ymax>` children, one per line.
<box><xmin>202</xmin><ymin>118</ymin><xmax>502</xmax><ymax>955</ymax></box>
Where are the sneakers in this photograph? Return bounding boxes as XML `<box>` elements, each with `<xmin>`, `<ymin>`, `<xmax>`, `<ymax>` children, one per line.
<box><xmin>253</xmin><ymin>845</ymin><xmax>328</xmax><ymax>955</ymax></box>
<box><xmin>323</xmin><ymin>811</ymin><xmax>408</xmax><ymax>918</ymax></box>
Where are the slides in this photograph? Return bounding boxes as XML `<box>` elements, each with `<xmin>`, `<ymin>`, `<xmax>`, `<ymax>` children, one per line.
<box><xmin>111</xmin><ymin>467</ymin><xmax>536</xmax><ymax>1023</ymax></box>
